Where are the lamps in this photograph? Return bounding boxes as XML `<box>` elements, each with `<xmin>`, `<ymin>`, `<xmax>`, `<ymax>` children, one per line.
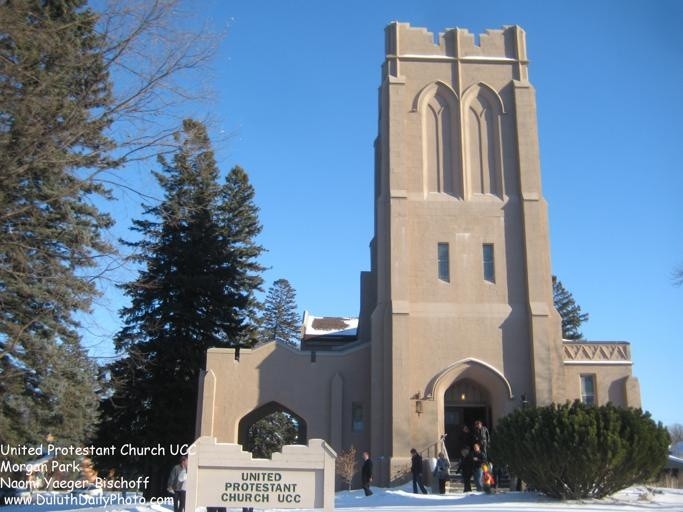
<box><xmin>415</xmin><ymin>395</ymin><xmax>423</xmax><ymax>417</ymax></box>
<box><xmin>520</xmin><ymin>393</ymin><xmax>528</xmax><ymax>408</ymax></box>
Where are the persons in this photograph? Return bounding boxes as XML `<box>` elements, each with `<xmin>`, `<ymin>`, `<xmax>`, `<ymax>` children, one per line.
<box><xmin>455</xmin><ymin>419</ymin><xmax>495</xmax><ymax>496</ymax></box>
<box><xmin>432</xmin><ymin>453</ymin><xmax>451</xmax><ymax>495</ymax></box>
<box><xmin>361</xmin><ymin>451</ymin><xmax>373</xmax><ymax>496</ymax></box>
<box><xmin>165</xmin><ymin>453</ymin><xmax>188</xmax><ymax>512</ymax></box>
<box><xmin>410</xmin><ymin>448</ymin><xmax>428</xmax><ymax>494</ymax></box>
<box><xmin>208</xmin><ymin>507</ymin><xmax>254</xmax><ymax>512</ymax></box>
